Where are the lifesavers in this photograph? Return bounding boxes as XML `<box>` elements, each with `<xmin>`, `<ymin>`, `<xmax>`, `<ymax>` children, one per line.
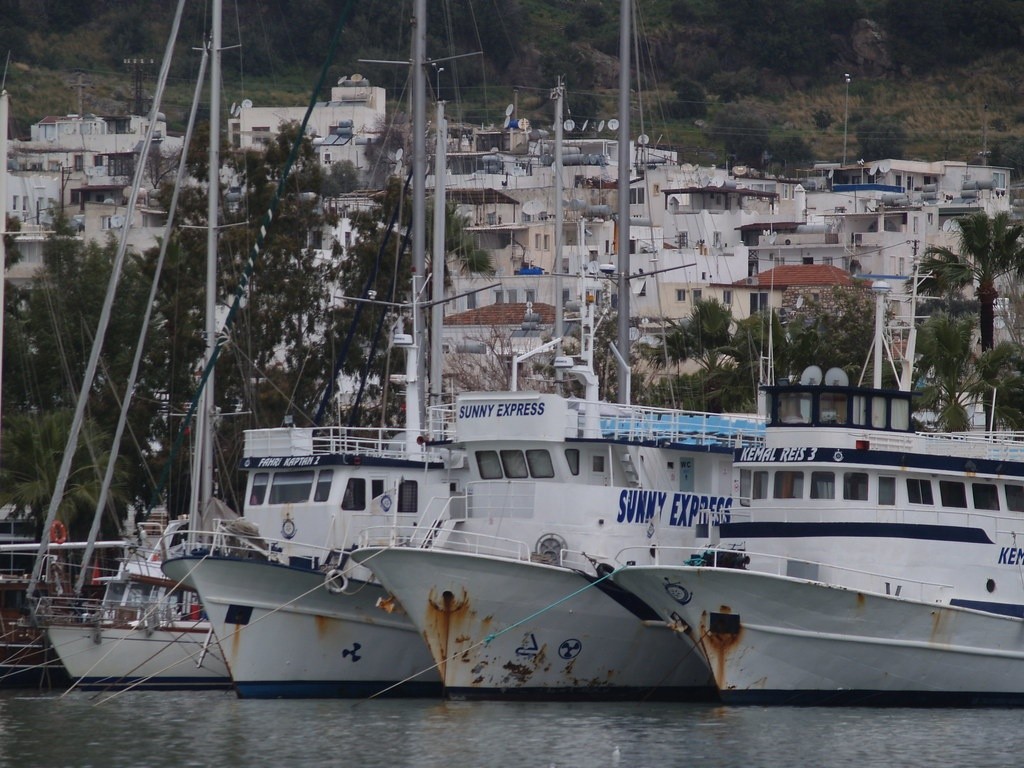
<box><xmin>50</xmin><ymin>520</ymin><xmax>67</xmax><ymax>544</ymax></box>
<box><xmin>325</xmin><ymin>570</ymin><xmax>349</xmax><ymax>594</ymax></box>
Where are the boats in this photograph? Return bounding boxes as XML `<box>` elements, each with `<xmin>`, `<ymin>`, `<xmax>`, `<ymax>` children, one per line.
<box><xmin>612</xmin><ymin>239</ymin><xmax>1024</xmax><ymax>706</ymax></box>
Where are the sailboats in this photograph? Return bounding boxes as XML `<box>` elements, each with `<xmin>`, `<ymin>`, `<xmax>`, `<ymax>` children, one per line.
<box><xmin>162</xmin><ymin>0</ymin><xmax>449</xmax><ymax>701</ymax></box>
<box><xmin>346</xmin><ymin>0</ymin><xmax>772</xmax><ymax>699</ymax></box>
<box><xmin>24</xmin><ymin>0</ymin><xmax>235</xmax><ymax>690</ymax></box>
